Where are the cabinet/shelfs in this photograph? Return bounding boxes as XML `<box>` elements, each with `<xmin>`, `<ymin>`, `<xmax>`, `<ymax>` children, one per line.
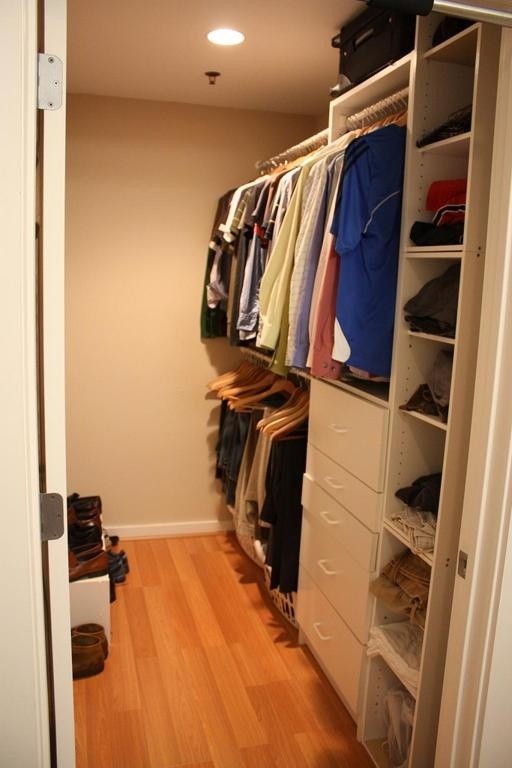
<box><xmin>293</xmin><ymin>376</ymin><xmax>390</xmax><ymax>743</ymax></box>
<box><xmin>68</xmin><ymin>511</ymin><xmax>111</xmax><ymax>647</ymax></box>
<box><xmin>357</xmin><ymin>7</ymin><xmax>500</xmax><ymax>768</ymax></box>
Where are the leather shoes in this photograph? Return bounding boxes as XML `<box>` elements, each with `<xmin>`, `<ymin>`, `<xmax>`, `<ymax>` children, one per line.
<box><xmin>67</xmin><ymin>493</ymin><xmax>128</xmax><ymax>602</ymax></box>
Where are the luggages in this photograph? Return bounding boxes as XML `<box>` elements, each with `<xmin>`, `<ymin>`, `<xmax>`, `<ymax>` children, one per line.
<box><xmin>329</xmin><ymin>6</ymin><xmax>415</xmax><ymax>98</ymax></box>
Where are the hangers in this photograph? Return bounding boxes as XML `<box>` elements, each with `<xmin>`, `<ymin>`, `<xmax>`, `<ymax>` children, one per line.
<box><xmin>230</xmin><ymin>88</ymin><xmax>407</xmax><ymax>185</ymax></box>
<box><xmin>206</xmin><ymin>347</ymin><xmax>313</xmax><ymax>443</ymax></box>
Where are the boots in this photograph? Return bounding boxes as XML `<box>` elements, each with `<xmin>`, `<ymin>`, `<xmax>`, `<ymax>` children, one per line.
<box><xmin>71</xmin><ymin>623</ymin><xmax>108</xmax><ymax>679</ymax></box>
